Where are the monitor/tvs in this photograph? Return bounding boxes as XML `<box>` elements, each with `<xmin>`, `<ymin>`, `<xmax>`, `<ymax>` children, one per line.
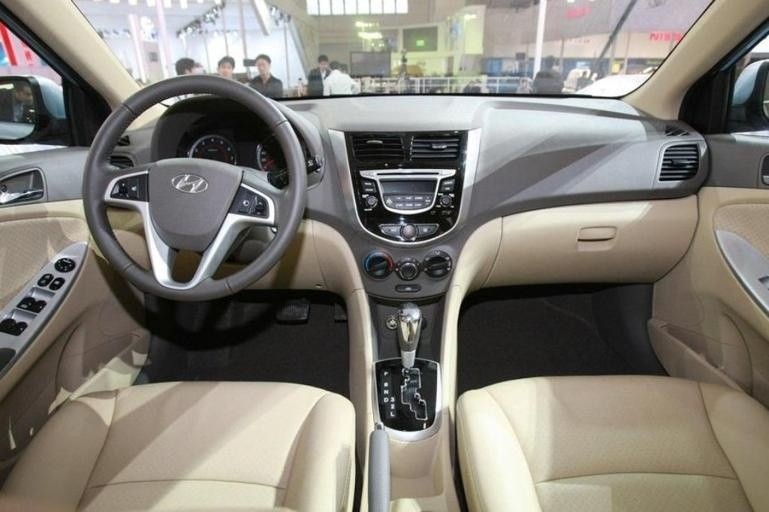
<box><xmin>349</xmin><ymin>51</ymin><xmax>390</xmax><ymax>78</ymax></box>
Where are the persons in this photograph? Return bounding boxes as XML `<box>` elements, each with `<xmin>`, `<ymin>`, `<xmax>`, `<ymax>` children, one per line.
<box><xmin>250</xmin><ymin>55</ymin><xmax>283</xmax><ymax>99</ymax></box>
<box><xmin>308</xmin><ymin>56</ymin><xmax>331</xmax><ymax>99</ymax></box>
<box><xmin>322</xmin><ymin>61</ymin><xmax>360</xmax><ymax>96</ymax></box>
<box><xmin>176</xmin><ymin>58</ymin><xmax>197</xmax><ymax>98</ymax></box>
<box><xmin>7</xmin><ymin>81</ymin><xmax>33</xmax><ymax>120</ymax></box>
<box><xmin>338</xmin><ymin>55</ymin><xmax>603</xmax><ymax>96</ymax></box>
<box><xmin>216</xmin><ymin>55</ymin><xmax>236</xmax><ymax>77</ymax></box>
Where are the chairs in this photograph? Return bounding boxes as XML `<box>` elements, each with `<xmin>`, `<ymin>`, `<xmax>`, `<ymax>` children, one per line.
<box><xmin>456</xmin><ymin>373</ymin><xmax>768</xmax><ymax>512</ymax></box>
<box><xmin>1</xmin><ymin>375</ymin><xmax>358</xmax><ymax>512</ymax></box>
